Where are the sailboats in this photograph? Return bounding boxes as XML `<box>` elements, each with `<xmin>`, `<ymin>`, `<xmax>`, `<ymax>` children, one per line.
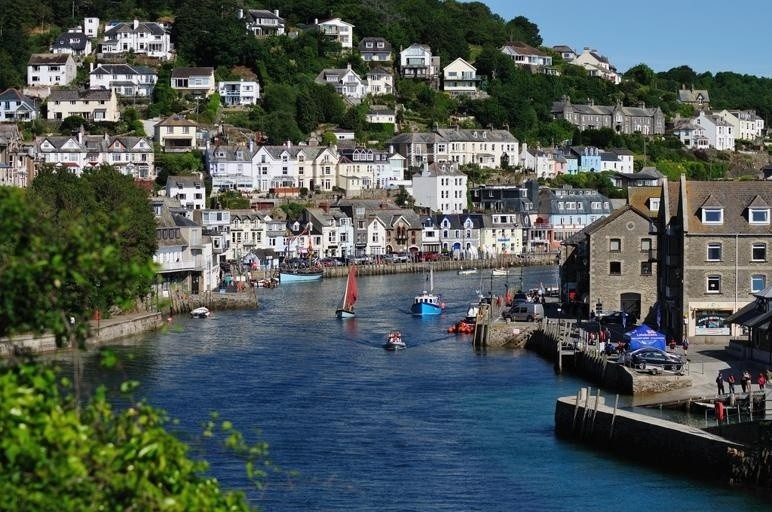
<box><xmin>336</xmin><ymin>264</ymin><xmax>358</xmax><ymax>318</ymax></box>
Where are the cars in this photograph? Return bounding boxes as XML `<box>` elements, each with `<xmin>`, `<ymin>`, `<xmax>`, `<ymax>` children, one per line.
<box><xmin>602</xmin><ymin>311</ymin><xmax>637</xmax><ymax>327</ymax></box>
<box><xmin>625</xmin><ymin>346</ymin><xmax>672</xmax><ymax>372</ymax></box>
<box><xmin>511</xmin><ymin>292</ymin><xmax>533</xmax><ymax>307</ymax></box>
<box><xmin>284</xmin><ymin>250</ymin><xmax>446</xmax><ymax>267</ymax></box>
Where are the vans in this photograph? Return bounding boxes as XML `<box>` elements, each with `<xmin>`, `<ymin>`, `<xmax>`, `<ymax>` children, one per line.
<box><xmin>501</xmin><ymin>303</ymin><xmax>544</xmax><ymax>323</ymax></box>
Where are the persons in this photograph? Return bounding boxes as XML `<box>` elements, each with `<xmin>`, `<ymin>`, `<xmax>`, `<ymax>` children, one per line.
<box><xmin>727</xmin><ymin>373</ymin><xmax>737</xmax><ymax>394</ymax></box>
<box><xmin>766</xmin><ymin>369</ymin><xmax>772</xmax><ymax>385</ymax></box>
<box><xmin>527</xmin><ymin>293</ymin><xmax>546</xmax><ymax>306</ymax></box>
<box><xmin>497</xmin><ymin>295</ymin><xmax>503</xmax><ymax>312</ymax></box>
<box><xmin>587</xmin><ymin>327</ymin><xmax>611</xmax><ymax>345</ymax></box>
<box><xmin>741</xmin><ymin>371</ymin><xmax>747</xmax><ymax>393</ymax></box>
<box><xmin>505</xmin><ymin>293</ymin><xmax>511</xmax><ymax>305</ymax></box>
<box><xmin>386</xmin><ymin>331</ymin><xmax>403</xmax><ymax>343</ymax></box>
<box><xmin>682</xmin><ymin>337</ymin><xmax>689</xmax><ymax>356</ymax></box>
<box><xmin>744</xmin><ymin>372</ymin><xmax>749</xmax><ymax>378</ymax></box>
<box><xmin>757</xmin><ymin>372</ymin><xmax>765</xmax><ymax>389</ymax></box>
<box><xmin>747</xmin><ymin>372</ymin><xmax>752</xmax><ymax>393</ymax></box>
<box><xmin>716</xmin><ymin>371</ymin><xmax>725</xmax><ymax>395</ymax></box>
<box><xmin>669</xmin><ymin>335</ymin><xmax>677</xmax><ymax>352</ymax></box>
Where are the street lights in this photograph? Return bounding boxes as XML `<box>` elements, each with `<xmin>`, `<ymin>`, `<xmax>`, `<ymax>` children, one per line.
<box><xmin>520</xmin><ymin>265</ymin><xmax>524</xmax><ymax>291</ymax></box>
<box><xmin>487</xmin><ymin>292</ymin><xmax>492</xmax><ymax>318</ymax></box>
<box><xmin>557</xmin><ymin>307</ymin><xmax>561</xmax><ymax>337</ymax></box>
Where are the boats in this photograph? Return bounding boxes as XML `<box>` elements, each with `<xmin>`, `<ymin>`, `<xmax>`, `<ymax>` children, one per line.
<box><xmin>190</xmin><ymin>306</ymin><xmax>210</xmax><ymax>318</ymax></box>
<box><xmin>384</xmin><ymin>330</ymin><xmax>407</xmax><ymax>351</ymax></box>
<box><xmin>279</xmin><ymin>273</ymin><xmax>323</xmax><ymax>283</ymax></box>
<box><xmin>491</xmin><ymin>267</ymin><xmax>510</xmax><ymax>276</ymax></box>
<box><xmin>410</xmin><ymin>290</ymin><xmax>446</xmax><ymax>317</ymax></box>
<box><xmin>465</xmin><ymin>295</ymin><xmax>499</xmax><ymax>324</ymax></box>
<box><xmin>457</xmin><ymin>266</ymin><xmax>478</xmax><ymax>275</ymax></box>
